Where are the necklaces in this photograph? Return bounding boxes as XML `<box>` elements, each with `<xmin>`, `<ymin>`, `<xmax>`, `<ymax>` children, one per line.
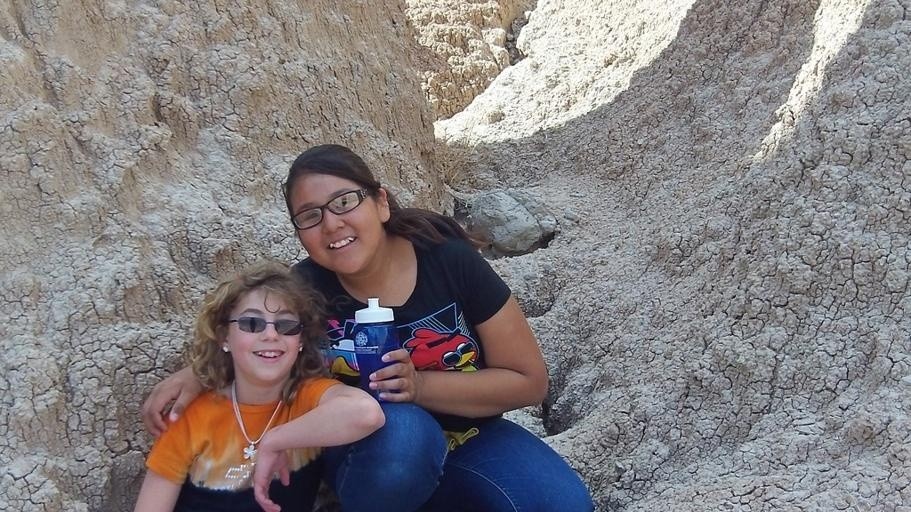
<box><xmin>229</xmin><ymin>377</ymin><xmax>283</xmax><ymax>459</ymax></box>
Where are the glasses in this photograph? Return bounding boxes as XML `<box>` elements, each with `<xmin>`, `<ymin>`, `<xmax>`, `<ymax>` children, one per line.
<box><xmin>230</xmin><ymin>318</ymin><xmax>303</xmax><ymax>335</ymax></box>
<box><xmin>290</xmin><ymin>188</ymin><xmax>370</xmax><ymax>230</ymax></box>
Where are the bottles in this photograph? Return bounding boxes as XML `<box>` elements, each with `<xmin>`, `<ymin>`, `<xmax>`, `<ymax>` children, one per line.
<box><xmin>356</xmin><ymin>294</ymin><xmax>401</xmax><ymax>407</ymax></box>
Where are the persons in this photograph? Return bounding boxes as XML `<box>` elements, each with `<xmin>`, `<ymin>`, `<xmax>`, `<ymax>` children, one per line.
<box><xmin>133</xmin><ymin>262</ymin><xmax>388</xmax><ymax>511</ymax></box>
<box><xmin>132</xmin><ymin>142</ymin><xmax>595</xmax><ymax>512</ymax></box>
